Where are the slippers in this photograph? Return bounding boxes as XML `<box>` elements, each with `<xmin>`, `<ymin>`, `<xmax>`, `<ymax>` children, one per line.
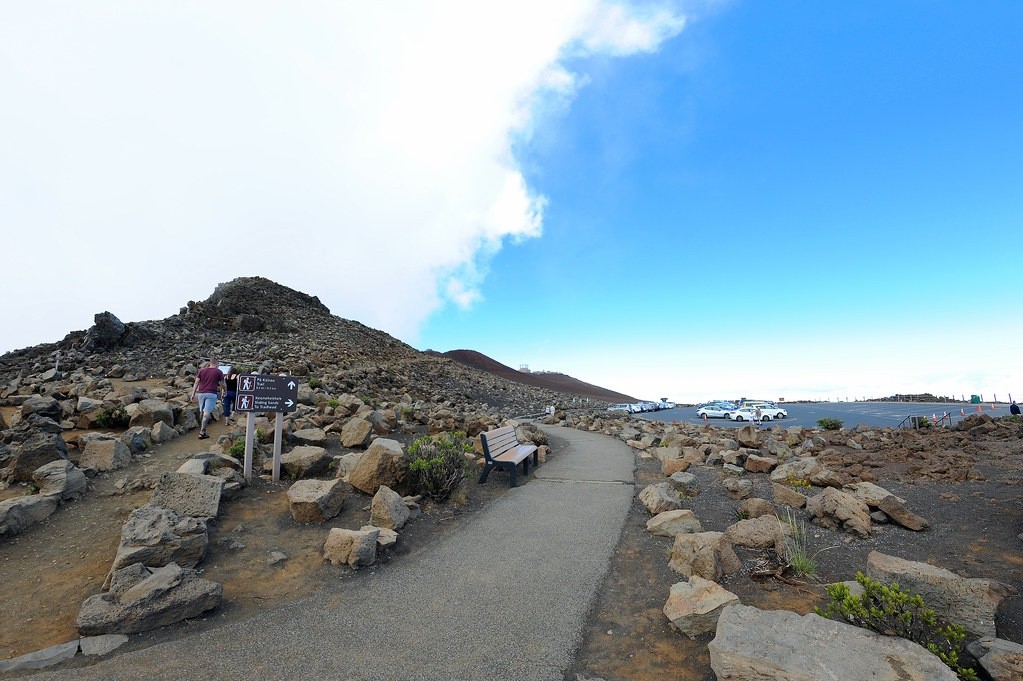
<box><xmin>225</xmin><ymin>422</ymin><xmax>230</xmax><ymax>425</ymax></box>
<box><xmin>230</xmin><ymin>417</ymin><xmax>235</xmax><ymax>422</ymax></box>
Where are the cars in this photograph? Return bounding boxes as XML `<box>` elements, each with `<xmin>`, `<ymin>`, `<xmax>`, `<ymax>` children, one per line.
<box><xmin>697</xmin><ymin>400</ymin><xmax>787</xmax><ymax>421</ymax></box>
<box><xmin>607</xmin><ymin>402</ymin><xmax>676</xmax><ymax>414</ymax></box>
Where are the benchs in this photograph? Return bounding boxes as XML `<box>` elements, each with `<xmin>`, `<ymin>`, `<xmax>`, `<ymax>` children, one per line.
<box><xmin>477</xmin><ymin>425</ymin><xmax>539</xmax><ymax>487</ymax></box>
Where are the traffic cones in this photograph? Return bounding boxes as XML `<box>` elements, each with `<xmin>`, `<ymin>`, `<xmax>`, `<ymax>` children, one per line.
<box><xmin>991</xmin><ymin>404</ymin><xmax>996</xmax><ymax>410</ymax></box>
<box><xmin>704</xmin><ymin>414</ymin><xmax>708</xmax><ymax>421</ymax></box>
<box><xmin>960</xmin><ymin>409</ymin><xmax>965</xmax><ymax>416</ymax></box>
<box><xmin>933</xmin><ymin>413</ymin><xmax>937</xmax><ymax>422</ymax></box>
<box><xmin>944</xmin><ymin>411</ymin><xmax>949</xmax><ymax>419</ymax></box>
<box><xmin>978</xmin><ymin>405</ymin><xmax>983</xmax><ymax>412</ymax></box>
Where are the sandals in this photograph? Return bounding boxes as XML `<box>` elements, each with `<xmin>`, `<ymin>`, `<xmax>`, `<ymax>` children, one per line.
<box><xmin>198</xmin><ymin>434</ymin><xmax>209</xmax><ymax>439</ymax></box>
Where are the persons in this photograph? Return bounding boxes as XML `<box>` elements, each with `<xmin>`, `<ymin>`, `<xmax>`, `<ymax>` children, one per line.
<box><xmin>222</xmin><ymin>368</ymin><xmax>240</xmax><ymax>426</ymax></box>
<box><xmin>191</xmin><ymin>357</ymin><xmax>227</xmax><ymax>440</ymax></box>
<box><xmin>1010</xmin><ymin>400</ymin><xmax>1021</xmax><ymax>415</ymax></box>
<box><xmin>545</xmin><ymin>405</ymin><xmax>555</xmax><ymax>416</ymax></box>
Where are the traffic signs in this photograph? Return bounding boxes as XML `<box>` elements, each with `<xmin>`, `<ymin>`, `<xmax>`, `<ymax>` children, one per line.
<box><xmin>235</xmin><ymin>373</ymin><xmax>298</xmax><ymax>412</ymax></box>
